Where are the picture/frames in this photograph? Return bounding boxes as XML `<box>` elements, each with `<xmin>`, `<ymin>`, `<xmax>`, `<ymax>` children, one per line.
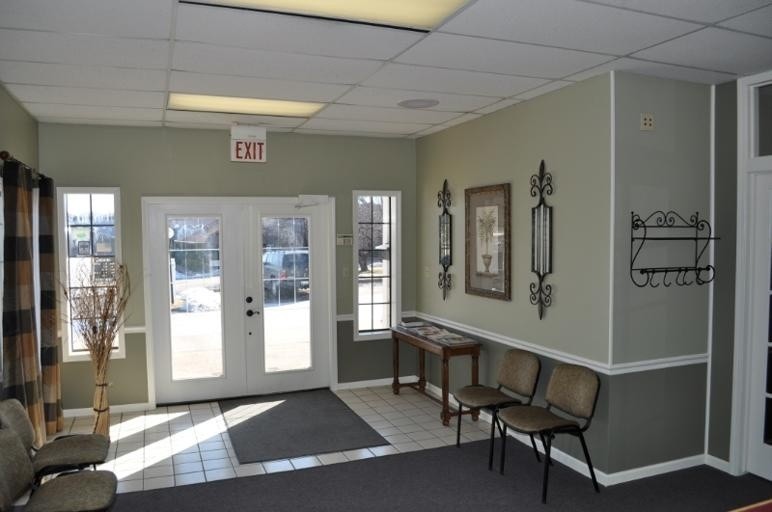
<box><xmin>464</xmin><ymin>182</ymin><xmax>511</xmax><ymax>301</ymax></box>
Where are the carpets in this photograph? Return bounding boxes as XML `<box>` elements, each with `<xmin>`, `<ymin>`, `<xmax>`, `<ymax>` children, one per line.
<box><xmin>218</xmin><ymin>388</ymin><xmax>389</xmax><ymax>463</ymax></box>
<box><xmin>107</xmin><ymin>434</ymin><xmax>772</xmax><ymax>512</ymax></box>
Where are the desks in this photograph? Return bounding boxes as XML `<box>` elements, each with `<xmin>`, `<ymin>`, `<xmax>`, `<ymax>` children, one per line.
<box><xmin>390</xmin><ymin>326</ymin><xmax>483</xmax><ymax>426</ymax></box>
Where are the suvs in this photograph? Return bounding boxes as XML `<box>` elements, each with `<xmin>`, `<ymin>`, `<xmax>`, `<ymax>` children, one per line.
<box><xmin>263</xmin><ymin>250</ymin><xmax>309</xmax><ymax>297</ymax></box>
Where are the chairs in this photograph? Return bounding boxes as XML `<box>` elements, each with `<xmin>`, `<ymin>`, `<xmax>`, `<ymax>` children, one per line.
<box><xmin>453</xmin><ymin>349</ymin><xmax>542</xmax><ymax>470</ymax></box>
<box><xmin>497</xmin><ymin>364</ymin><xmax>601</xmax><ymax>503</ymax></box>
<box><xmin>0</xmin><ymin>430</ymin><xmax>118</xmax><ymax>512</ymax></box>
<box><xmin>0</xmin><ymin>398</ymin><xmax>110</xmax><ymax>497</ymax></box>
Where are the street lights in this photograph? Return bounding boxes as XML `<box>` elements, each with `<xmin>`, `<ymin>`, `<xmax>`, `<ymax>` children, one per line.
<box><xmin>169</xmin><ymin>227</ymin><xmax>175</xmax><ymax>305</ymax></box>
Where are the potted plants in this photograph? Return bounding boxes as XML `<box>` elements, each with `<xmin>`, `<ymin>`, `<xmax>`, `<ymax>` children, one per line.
<box><xmin>477</xmin><ymin>208</ymin><xmax>496</xmax><ymax>272</ymax></box>
